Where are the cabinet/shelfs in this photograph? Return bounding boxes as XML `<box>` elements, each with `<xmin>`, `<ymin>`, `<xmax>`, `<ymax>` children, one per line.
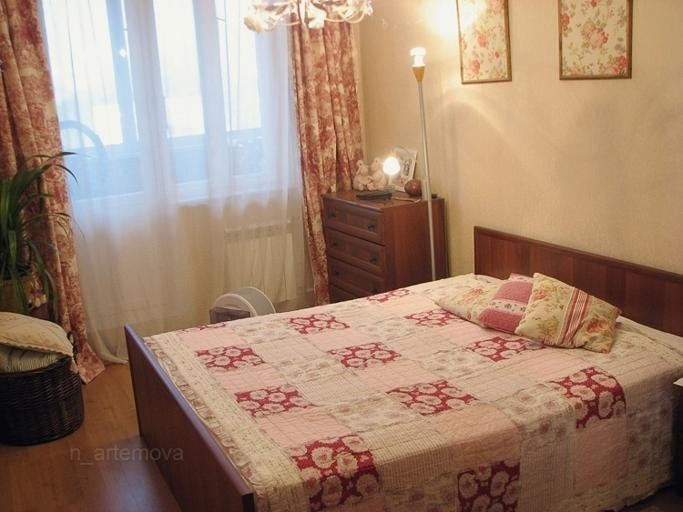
<box><xmin>319</xmin><ymin>189</ymin><xmax>448</xmax><ymax>304</ymax></box>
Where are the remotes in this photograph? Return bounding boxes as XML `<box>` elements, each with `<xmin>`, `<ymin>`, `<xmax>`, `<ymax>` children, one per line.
<box><xmin>356</xmin><ymin>192</ymin><xmax>392</xmax><ymax>200</ymax></box>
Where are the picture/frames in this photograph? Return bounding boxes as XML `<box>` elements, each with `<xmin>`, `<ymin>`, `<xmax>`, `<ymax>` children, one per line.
<box><xmin>455</xmin><ymin>0</ymin><xmax>513</xmax><ymax>85</ymax></box>
<box><xmin>556</xmin><ymin>1</ymin><xmax>633</xmax><ymax>82</ymax></box>
<box><xmin>388</xmin><ymin>145</ymin><xmax>420</xmax><ymax>194</ymax></box>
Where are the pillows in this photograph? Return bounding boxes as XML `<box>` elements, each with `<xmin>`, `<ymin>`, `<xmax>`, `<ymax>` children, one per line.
<box><xmin>438</xmin><ymin>273</ymin><xmax>624</xmax><ymax>353</ymax></box>
<box><xmin>0</xmin><ymin>308</ymin><xmax>76</xmax><ymax>362</ymax></box>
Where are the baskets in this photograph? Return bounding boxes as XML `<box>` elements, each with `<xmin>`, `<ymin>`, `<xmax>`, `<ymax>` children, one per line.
<box><xmin>0</xmin><ymin>330</ymin><xmax>84</xmax><ymax>446</ymax></box>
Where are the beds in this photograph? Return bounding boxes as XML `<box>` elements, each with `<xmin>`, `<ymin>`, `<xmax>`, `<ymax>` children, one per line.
<box><xmin>123</xmin><ymin>220</ymin><xmax>683</xmax><ymax>510</ymax></box>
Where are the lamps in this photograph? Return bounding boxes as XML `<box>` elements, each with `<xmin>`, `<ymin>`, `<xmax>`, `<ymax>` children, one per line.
<box><xmin>241</xmin><ymin>1</ymin><xmax>376</xmax><ymax>33</ymax></box>
<box><xmin>410</xmin><ymin>46</ymin><xmax>439</xmax><ymax>281</ymax></box>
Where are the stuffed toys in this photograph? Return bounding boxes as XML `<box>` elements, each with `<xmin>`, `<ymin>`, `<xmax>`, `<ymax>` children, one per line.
<box><xmin>353</xmin><ymin>159</ymin><xmax>372</xmax><ymax>191</ymax></box>
<box><xmin>365</xmin><ymin>157</ymin><xmax>387</xmax><ymax>190</ymax></box>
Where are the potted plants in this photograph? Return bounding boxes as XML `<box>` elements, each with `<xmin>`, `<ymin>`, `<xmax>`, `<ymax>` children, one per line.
<box><xmin>0</xmin><ymin>149</ymin><xmax>88</xmax><ymax>307</ymax></box>
